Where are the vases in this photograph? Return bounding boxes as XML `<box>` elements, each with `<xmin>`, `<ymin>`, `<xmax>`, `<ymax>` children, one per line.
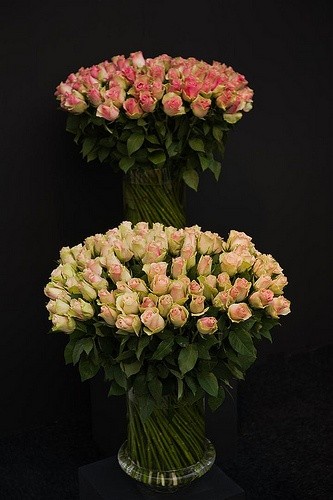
<box><xmin>119</xmin><ymin>168</ymin><xmax>188</xmax><ymax>231</ymax></box>
<box><xmin>115</xmin><ymin>387</ymin><xmax>216</xmax><ymax>487</ymax></box>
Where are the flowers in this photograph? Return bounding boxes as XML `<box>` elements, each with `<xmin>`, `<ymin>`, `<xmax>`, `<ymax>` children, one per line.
<box><xmin>54</xmin><ymin>50</ymin><xmax>256</xmax><ymax>227</ymax></box>
<box><xmin>42</xmin><ymin>219</ymin><xmax>293</xmax><ymax>474</ymax></box>
